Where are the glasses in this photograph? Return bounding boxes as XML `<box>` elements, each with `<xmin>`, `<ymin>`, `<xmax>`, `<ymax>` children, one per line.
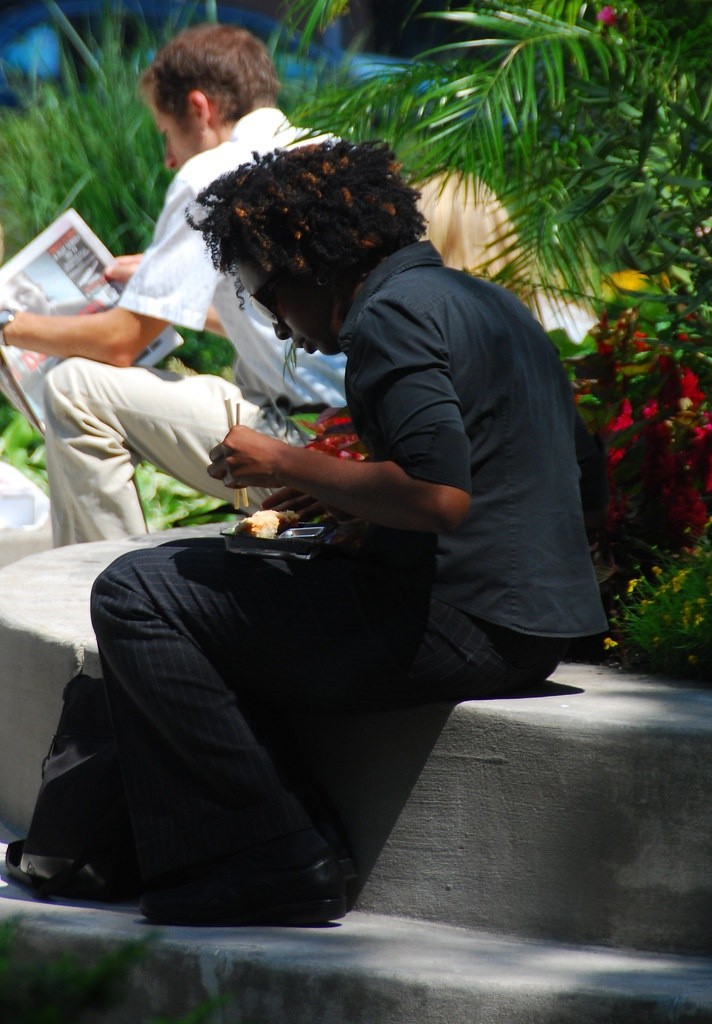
<box><xmin>248</xmin><ymin>266</ymin><xmax>287</xmax><ymax>325</ymax></box>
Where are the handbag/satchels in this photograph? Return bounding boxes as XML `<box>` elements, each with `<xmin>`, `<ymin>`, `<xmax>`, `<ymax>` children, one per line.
<box><xmin>6</xmin><ymin>675</ymin><xmax>191</xmax><ymax>904</ymax></box>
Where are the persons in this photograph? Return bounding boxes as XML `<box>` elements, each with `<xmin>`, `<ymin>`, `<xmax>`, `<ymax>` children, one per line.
<box><xmin>1</xmin><ymin>26</ymin><xmax>350</xmax><ymax>548</ymax></box>
<box><xmin>4</xmin><ymin>139</ymin><xmax>610</xmax><ymax>925</ymax></box>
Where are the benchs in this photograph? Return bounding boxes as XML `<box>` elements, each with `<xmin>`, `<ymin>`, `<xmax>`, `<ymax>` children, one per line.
<box><xmin>1</xmin><ymin>520</ymin><xmax>712</xmax><ymax>956</ymax></box>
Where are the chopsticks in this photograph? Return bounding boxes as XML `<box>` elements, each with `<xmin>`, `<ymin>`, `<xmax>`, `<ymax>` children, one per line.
<box><xmin>224</xmin><ymin>398</ymin><xmax>249</xmax><ymax>510</ymax></box>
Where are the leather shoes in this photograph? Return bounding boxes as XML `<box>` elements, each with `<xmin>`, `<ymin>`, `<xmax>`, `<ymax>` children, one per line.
<box><xmin>139</xmin><ymin>857</ymin><xmax>360</xmax><ymax>928</ymax></box>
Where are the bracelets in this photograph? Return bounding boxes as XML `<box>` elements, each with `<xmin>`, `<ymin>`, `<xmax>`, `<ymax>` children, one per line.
<box><xmin>1</xmin><ymin>309</ymin><xmax>9</xmax><ymax>344</ymax></box>
